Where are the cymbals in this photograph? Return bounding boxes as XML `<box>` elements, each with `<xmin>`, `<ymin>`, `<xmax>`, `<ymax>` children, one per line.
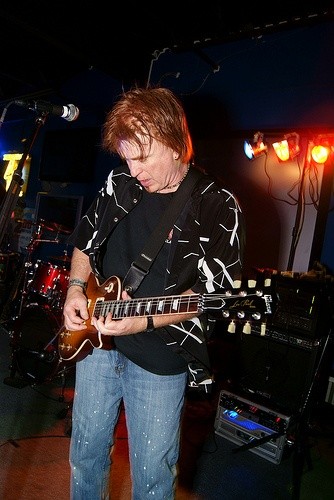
<box><xmin>40</xmin><ymin>220</ymin><xmax>73</xmax><ymax>234</ymax></box>
<box><xmin>49</xmin><ymin>255</ymin><xmax>71</xmax><ymax>262</ymax></box>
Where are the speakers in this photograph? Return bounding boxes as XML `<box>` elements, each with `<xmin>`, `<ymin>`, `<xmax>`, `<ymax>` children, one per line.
<box><xmin>228</xmin><ymin>334</ymin><xmax>318</xmax><ymax>417</ymax></box>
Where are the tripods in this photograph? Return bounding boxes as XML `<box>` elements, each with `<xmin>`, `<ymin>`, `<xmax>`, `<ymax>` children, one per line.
<box><xmin>235</xmin><ymin>324</ymin><xmax>333</xmax><ymax>500</ymax></box>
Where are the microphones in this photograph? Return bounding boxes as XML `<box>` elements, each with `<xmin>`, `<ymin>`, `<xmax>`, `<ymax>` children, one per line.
<box><xmin>14</xmin><ymin>99</ymin><xmax>79</xmax><ymax>122</ymax></box>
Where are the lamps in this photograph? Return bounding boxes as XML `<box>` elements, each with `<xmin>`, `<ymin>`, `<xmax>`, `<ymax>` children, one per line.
<box><xmin>242</xmin><ymin>132</ymin><xmax>334</xmax><ymax>165</ymax></box>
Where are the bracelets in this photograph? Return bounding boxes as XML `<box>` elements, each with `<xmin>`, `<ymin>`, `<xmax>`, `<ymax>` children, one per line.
<box><xmin>143</xmin><ymin>316</ymin><xmax>155</xmax><ymax>332</ymax></box>
<box><xmin>67</xmin><ymin>279</ymin><xmax>86</xmax><ymax>290</ymax></box>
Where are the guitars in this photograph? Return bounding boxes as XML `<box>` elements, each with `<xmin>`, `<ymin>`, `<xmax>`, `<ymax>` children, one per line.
<box><xmin>58</xmin><ymin>272</ymin><xmax>280</xmax><ymax>361</ymax></box>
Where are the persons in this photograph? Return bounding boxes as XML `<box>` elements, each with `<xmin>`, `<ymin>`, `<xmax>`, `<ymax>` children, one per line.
<box><xmin>63</xmin><ymin>88</ymin><xmax>241</xmax><ymax>500</ymax></box>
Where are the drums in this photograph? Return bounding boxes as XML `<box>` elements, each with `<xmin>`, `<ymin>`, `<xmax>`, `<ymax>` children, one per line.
<box><xmin>32</xmin><ymin>262</ymin><xmax>70</xmax><ymax>299</ymax></box>
